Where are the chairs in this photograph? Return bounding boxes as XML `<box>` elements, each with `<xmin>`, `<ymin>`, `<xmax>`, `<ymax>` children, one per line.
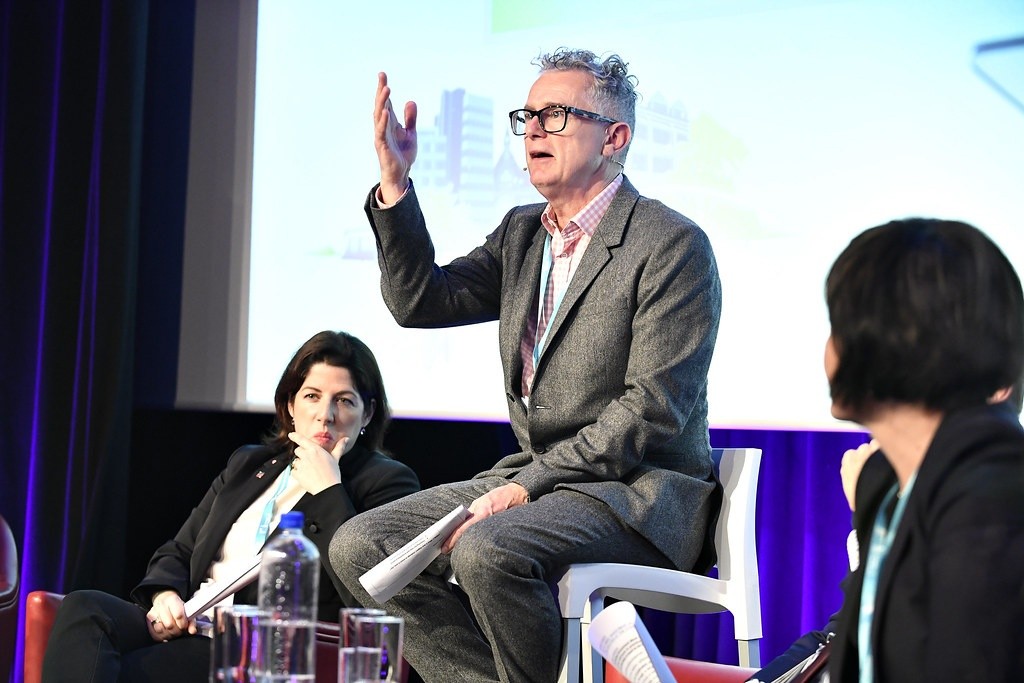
<box><xmin>554</xmin><ymin>445</ymin><xmax>763</xmax><ymax>683</ymax></box>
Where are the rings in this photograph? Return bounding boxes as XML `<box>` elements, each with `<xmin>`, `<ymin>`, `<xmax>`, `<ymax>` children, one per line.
<box><xmin>151</xmin><ymin>619</ymin><xmax>163</xmax><ymax>626</ymax></box>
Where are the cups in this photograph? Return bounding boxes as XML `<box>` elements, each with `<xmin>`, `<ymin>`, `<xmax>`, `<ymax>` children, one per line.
<box><xmin>358</xmin><ymin>618</ymin><xmax>404</xmax><ymax>683</ymax></box>
<box><xmin>225</xmin><ymin>611</ymin><xmax>270</xmax><ymax>683</ymax></box>
<box><xmin>338</xmin><ymin>609</ymin><xmax>387</xmax><ymax>683</ymax></box>
<box><xmin>214</xmin><ymin>606</ymin><xmax>259</xmax><ymax>683</ymax></box>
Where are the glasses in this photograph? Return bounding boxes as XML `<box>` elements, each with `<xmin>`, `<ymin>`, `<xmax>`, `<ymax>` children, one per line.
<box><xmin>509</xmin><ymin>106</ymin><xmax>618</xmax><ymax>137</ymax></box>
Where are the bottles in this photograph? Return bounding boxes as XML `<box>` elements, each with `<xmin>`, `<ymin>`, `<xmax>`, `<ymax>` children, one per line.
<box><xmin>257</xmin><ymin>512</ymin><xmax>320</xmax><ymax>683</ymax></box>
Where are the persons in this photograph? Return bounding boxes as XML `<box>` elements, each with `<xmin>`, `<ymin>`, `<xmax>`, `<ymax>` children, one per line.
<box><xmin>329</xmin><ymin>47</ymin><xmax>722</xmax><ymax>683</ymax></box>
<box><xmin>745</xmin><ymin>217</ymin><xmax>1024</xmax><ymax>683</ymax></box>
<box><xmin>42</xmin><ymin>331</ymin><xmax>420</xmax><ymax>683</ymax></box>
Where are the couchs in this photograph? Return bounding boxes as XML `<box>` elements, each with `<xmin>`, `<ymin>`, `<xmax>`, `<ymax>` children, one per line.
<box><xmin>24</xmin><ymin>592</ymin><xmax>423</xmax><ymax>683</ymax></box>
